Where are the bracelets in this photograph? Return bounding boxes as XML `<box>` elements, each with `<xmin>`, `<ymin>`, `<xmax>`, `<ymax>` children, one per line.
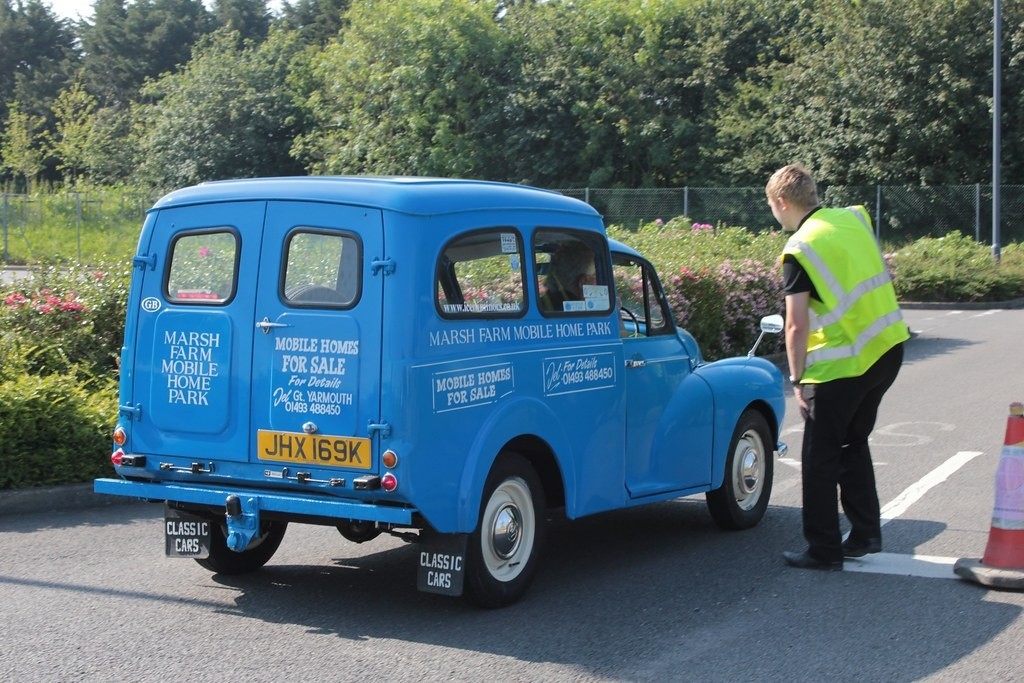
<box><xmin>788</xmin><ymin>377</ymin><xmax>802</xmax><ymax>386</ymax></box>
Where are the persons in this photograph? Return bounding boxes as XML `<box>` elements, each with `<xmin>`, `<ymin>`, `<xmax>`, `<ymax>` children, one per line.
<box><xmin>765</xmin><ymin>165</ymin><xmax>911</xmax><ymax>572</ymax></box>
<box><xmin>542</xmin><ymin>245</ymin><xmax>626</xmax><ymax>331</ymax></box>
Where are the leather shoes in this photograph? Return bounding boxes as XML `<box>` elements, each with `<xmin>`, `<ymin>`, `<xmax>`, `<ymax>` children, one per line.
<box><xmin>780</xmin><ymin>548</ymin><xmax>843</xmax><ymax>571</ymax></box>
<box><xmin>841</xmin><ymin>538</ymin><xmax>882</xmax><ymax>557</ymax></box>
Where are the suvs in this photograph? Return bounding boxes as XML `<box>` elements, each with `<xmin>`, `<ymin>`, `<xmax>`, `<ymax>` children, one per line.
<box><xmin>93</xmin><ymin>174</ymin><xmax>793</xmax><ymax>611</ymax></box>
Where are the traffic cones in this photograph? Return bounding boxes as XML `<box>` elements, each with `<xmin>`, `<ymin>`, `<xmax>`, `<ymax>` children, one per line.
<box><xmin>952</xmin><ymin>402</ymin><xmax>1024</xmax><ymax>592</ymax></box>
